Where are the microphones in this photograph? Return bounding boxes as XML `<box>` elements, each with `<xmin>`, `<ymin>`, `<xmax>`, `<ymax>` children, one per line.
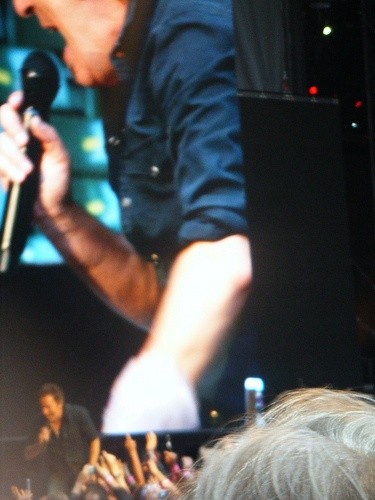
<box><xmin>0</xmin><ymin>51</ymin><xmax>61</xmax><ymax>282</ymax></box>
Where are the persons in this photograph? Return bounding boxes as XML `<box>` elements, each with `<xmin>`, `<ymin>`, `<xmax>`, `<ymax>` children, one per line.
<box><xmin>19</xmin><ymin>382</ymin><xmax>100</xmax><ymax>496</ymax></box>
<box><xmin>0</xmin><ymin>430</ymin><xmax>201</xmax><ymax>500</ymax></box>
<box><xmin>164</xmin><ymin>388</ymin><xmax>374</xmax><ymax>500</ymax></box>
<box><xmin>0</xmin><ymin>0</ymin><xmax>254</xmax><ymax>435</ymax></box>
